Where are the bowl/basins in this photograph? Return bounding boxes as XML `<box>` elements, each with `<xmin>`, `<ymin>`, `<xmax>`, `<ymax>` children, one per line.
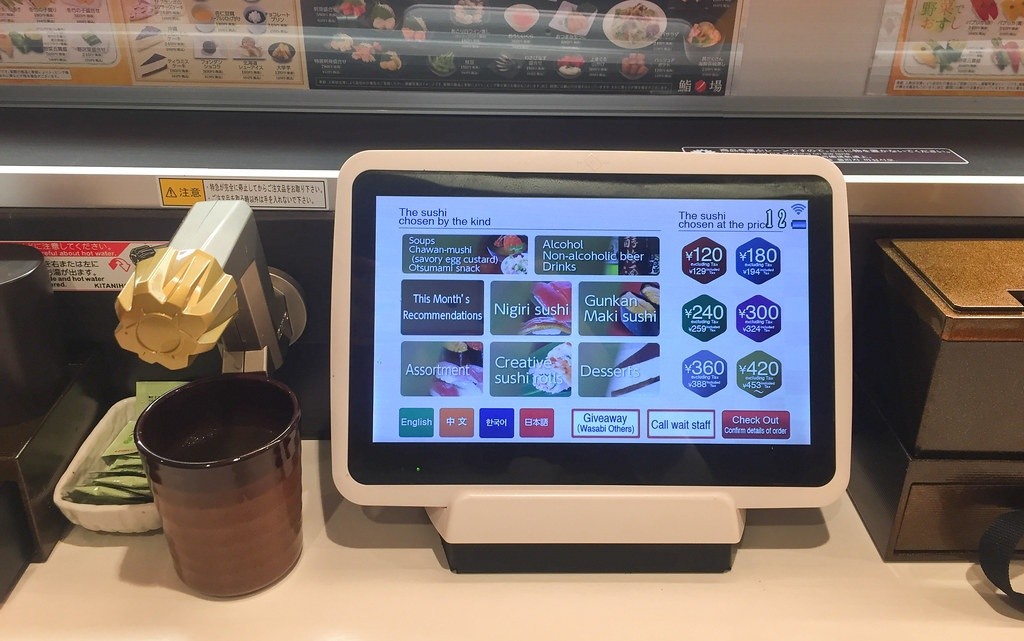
<box><xmin>441</xmin><ymin>342</ymin><xmax>468</xmax><ymax>365</ymax></box>
<box><xmin>466</xmin><ymin>343</ymin><xmax>483</xmax><ymax>368</ymax></box>
<box><xmin>485</xmin><ymin>235</ymin><xmax>527</xmax><ymax>262</ymax></box>
<box><xmin>641</xmin><ymin>280</ymin><xmax>659</xmax><ymax>311</ymax></box>
<box><xmin>620</xmin><ymin>290</ymin><xmax>659</xmax><ymax>335</ymax></box>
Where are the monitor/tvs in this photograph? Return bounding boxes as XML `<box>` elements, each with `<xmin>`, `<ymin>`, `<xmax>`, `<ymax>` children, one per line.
<box><xmin>331</xmin><ymin>148</ymin><xmax>854</xmax><ymax>504</ymax></box>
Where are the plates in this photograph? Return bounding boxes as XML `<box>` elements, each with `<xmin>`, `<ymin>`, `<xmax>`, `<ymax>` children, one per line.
<box><xmin>501</xmin><ymin>252</ymin><xmax>527</xmax><ymax>275</ymax></box>
<box><xmin>233</xmin><ymin>40</ymin><xmax>267</xmax><ymax>60</ymax></box>
<box><xmin>324</xmin><ymin>0</ymin><xmax>726</xmax><ymax>79</ymax></box>
<box><xmin>53</xmin><ymin>396</ymin><xmax>162</xmax><ymax>532</ymax></box>
<box><xmin>194</xmin><ymin>38</ymin><xmax>227</xmax><ymax>59</ymax></box>
<box><xmin>430</xmin><ymin>364</ymin><xmax>483</xmax><ymax>395</ymax></box>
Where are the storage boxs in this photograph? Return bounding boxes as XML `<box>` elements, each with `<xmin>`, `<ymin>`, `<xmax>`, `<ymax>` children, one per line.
<box><xmin>847</xmin><ymin>231</ymin><xmax>1024</xmax><ymax>564</ymax></box>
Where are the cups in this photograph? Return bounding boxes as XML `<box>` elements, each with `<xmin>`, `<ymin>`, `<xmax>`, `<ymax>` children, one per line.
<box><xmin>190</xmin><ymin>3</ymin><xmax>216</xmax><ymax>32</ymax></box>
<box><xmin>243</xmin><ymin>6</ymin><xmax>268</xmax><ymax>34</ymax></box>
<box><xmin>1</xmin><ymin>244</ymin><xmax>65</xmax><ymax>429</ymax></box>
<box><xmin>266</xmin><ymin>42</ymin><xmax>296</xmax><ymax>62</ymax></box>
<box><xmin>133</xmin><ymin>372</ymin><xmax>306</xmax><ymax>599</ymax></box>
